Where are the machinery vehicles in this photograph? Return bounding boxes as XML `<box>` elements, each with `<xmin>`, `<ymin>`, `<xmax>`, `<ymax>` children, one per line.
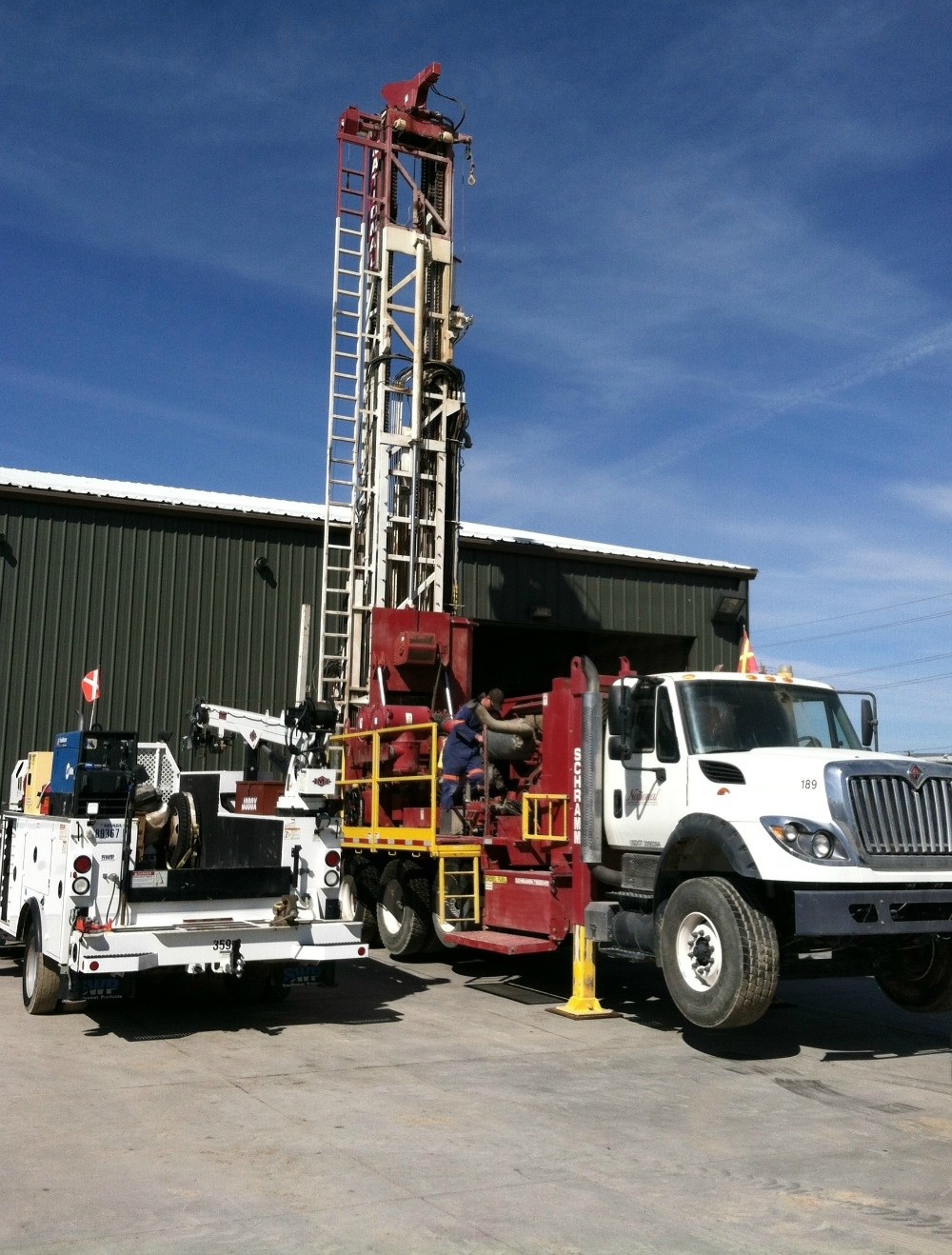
<box><xmin>290</xmin><ymin>58</ymin><xmax>952</xmax><ymax>1035</ymax></box>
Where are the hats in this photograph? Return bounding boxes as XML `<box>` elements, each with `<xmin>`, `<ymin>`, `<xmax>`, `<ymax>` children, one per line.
<box><xmin>490</xmin><ymin>689</ymin><xmax>504</xmax><ymax>710</ymax></box>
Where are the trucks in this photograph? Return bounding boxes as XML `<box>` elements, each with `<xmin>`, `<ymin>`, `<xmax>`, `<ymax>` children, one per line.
<box><xmin>0</xmin><ymin>667</ymin><xmax>375</xmax><ymax>1018</ymax></box>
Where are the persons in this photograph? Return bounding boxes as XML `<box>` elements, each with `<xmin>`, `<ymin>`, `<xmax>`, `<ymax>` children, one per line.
<box><xmin>442</xmin><ymin>688</ymin><xmax>504</xmax><ymax>809</ymax></box>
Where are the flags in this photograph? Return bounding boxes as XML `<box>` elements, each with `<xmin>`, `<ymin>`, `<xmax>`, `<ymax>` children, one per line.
<box><xmin>736</xmin><ymin>631</ymin><xmax>759</xmax><ymax>674</ymax></box>
<box><xmin>81</xmin><ymin>670</ymin><xmax>100</xmax><ymax>703</ymax></box>
